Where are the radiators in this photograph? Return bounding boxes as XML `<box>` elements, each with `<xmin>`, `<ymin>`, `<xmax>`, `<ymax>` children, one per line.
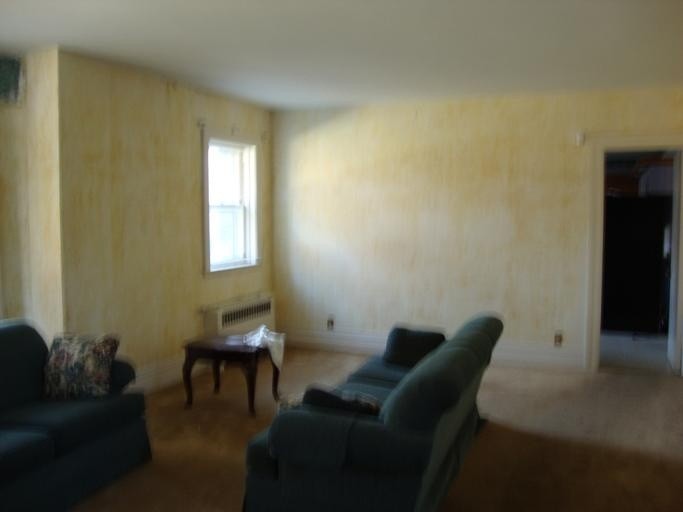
<box><xmin>204</xmin><ymin>294</ymin><xmax>277</xmax><ymax>376</ymax></box>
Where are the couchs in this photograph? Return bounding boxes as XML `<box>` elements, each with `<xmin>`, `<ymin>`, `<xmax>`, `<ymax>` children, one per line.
<box><xmin>0</xmin><ymin>318</ymin><xmax>153</xmax><ymax>512</ymax></box>
<box><xmin>246</xmin><ymin>315</ymin><xmax>505</xmax><ymax>512</ymax></box>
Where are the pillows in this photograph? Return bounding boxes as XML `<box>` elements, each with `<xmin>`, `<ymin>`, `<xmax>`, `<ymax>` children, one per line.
<box><xmin>304</xmin><ymin>383</ymin><xmax>380</xmax><ymax>417</ymax></box>
<box><xmin>41</xmin><ymin>333</ymin><xmax>119</xmax><ymax>402</ymax></box>
